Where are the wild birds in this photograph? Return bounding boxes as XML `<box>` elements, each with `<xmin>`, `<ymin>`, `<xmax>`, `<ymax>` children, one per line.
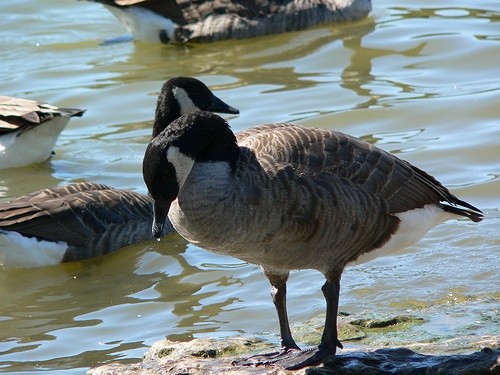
<box><xmin>92</xmin><ymin>0</ymin><xmax>372</xmax><ymax>44</ymax></box>
<box><xmin>0</xmin><ymin>78</ymin><xmax>239</xmax><ymax>266</ymax></box>
<box><xmin>143</xmin><ymin>111</ymin><xmax>483</xmax><ymax>369</ymax></box>
